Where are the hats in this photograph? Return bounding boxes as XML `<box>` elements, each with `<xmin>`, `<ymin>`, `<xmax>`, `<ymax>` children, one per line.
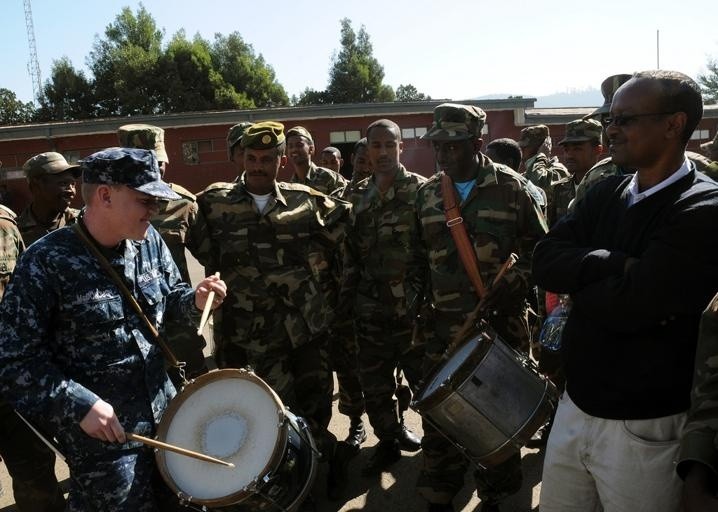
<box><xmin>22</xmin><ymin>151</ymin><xmax>82</xmax><ymax>184</ymax></box>
<box><xmin>241</xmin><ymin>120</ymin><xmax>286</xmax><ymax>150</ymax></box>
<box><xmin>581</xmin><ymin>74</ymin><xmax>634</xmax><ymax>121</ymax></box>
<box><xmin>285</xmin><ymin>125</ymin><xmax>314</xmax><ymax>142</ymax></box>
<box><xmin>117</xmin><ymin>123</ymin><xmax>170</xmax><ymax>165</ymax></box>
<box><xmin>557</xmin><ymin>118</ymin><xmax>603</xmax><ymax>146</ymax></box>
<box><xmin>225</xmin><ymin>122</ymin><xmax>256</xmax><ymax>148</ymax></box>
<box><xmin>418</xmin><ymin>102</ymin><xmax>487</xmax><ymax>142</ymax></box>
<box><xmin>517</xmin><ymin>124</ymin><xmax>551</xmax><ymax>149</ymax></box>
<box><xmin>79</xmin><ymin>146</ymin><xmax>183</xmax><ymax>201</ymax></box>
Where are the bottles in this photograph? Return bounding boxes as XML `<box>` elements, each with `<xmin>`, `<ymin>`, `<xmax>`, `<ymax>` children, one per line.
<box><xmin>538</xmin><ymin>292</ymin><xmax>574</xmax><ymax>351</ymax></box>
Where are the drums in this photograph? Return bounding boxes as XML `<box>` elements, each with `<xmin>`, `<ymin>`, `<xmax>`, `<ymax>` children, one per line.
<box><xmin>152</xmin><ymin>366</ymin><xmax>320</xmax><ymax>510</ymax></box>
<box><xmin>409</xmin><ymin>323</ymin><xmax>560</xmax><ymax>472</ymax></box>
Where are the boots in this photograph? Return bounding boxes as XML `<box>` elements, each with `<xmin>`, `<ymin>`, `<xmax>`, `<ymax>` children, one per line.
<box><xmin>337</xmin><ymin>419</ymin><xmax>367</xmax><ymax>459</ymax></box>
<box><xmin>379</xmin><ymin>423</ymin><xmax>421</xmax><ymax>452</ymax></box>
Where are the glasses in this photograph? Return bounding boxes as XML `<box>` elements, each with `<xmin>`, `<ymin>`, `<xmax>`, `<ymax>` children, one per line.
<box><xmin>602</xmin><ymin>111</ymin><xmax>676</xmax><ymax>126</ymax></box>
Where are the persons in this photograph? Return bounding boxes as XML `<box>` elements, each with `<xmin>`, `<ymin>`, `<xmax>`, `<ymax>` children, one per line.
<box><xmin>199</xmin><ymin>117</ymin><xmax>358</xmax><ymax>457</ymax></box>
<box><xmin>565</xmin><ymin>72</ymin><xmax>718</xmax><ymax>217</ymax></box>
<box><xmin>18</xmin><ymin>150</ymin><xmax>84</xmax><ymax>249</ymax></box>
<box><xmin>224</xmin><ymin>120</ymin><xmax>253</xmax><ymax>183</ymax></box>
<box><xmin>680</xmin><ymin>292</ymin><xmax>716</xmax><ymax>508</ymax></box>
<box><xmin>548</xmin><ymin>116</ymin><xmax>605</xmax><ymax>232</ymax></box>
<box><xmin>330</xmin><ymin>137</ymin><xmax>421</xmax><ymax>452</ymax></box>
<box><xmin>519</xmin><ymin>123</ymin><xmax>571</xmax><ymax>190</ymax></box>
<box><xmin>285</xmin><ymin>126</ymin><xmax>348</xmax><ymax>200</ymax></box>
<box><xmin>319</xmin><ymin>144</ymin><xmax>344</xmax><ymax>175</ymax></box>
<box><xmin>528</xmin><ymin>67</ymin><xmax>718</xmax><ymax>512</ymax></box>
<box><xmin>0</xmin><ymin>143</ymin><xmax>230</xmax><ymax>512</ymax></box>
<box><xmin>0</xmin><ymin>203</ymin><xmax>69</xmax><ymax>512</ymax></box>
<box><xmin>345</xmin><ymin>119</ymin><xmax>432</xmax><ymax>468</ymax></box>
<box><xmin>111</xmin><ymin>120</ymin><xmax>211</xmax><ymax>379</ymax></box>
<box><xmin>413</xmin><ymin>101</ymin><xmax>547</xmax><ymax>508</ymax></box>
<box><xmin>484</xmin><ymin>138</ymin><xmax>548</xmax><ymax>222</ymax></box>
